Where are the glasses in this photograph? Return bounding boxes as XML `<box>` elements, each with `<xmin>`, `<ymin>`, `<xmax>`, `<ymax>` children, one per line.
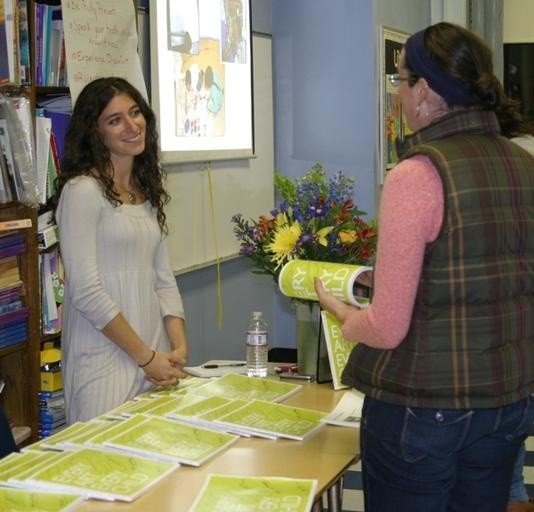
<box><xmin>390</xmin><ymin>75</ymin><xmax>417</xmax><ymax>85</ymax></box>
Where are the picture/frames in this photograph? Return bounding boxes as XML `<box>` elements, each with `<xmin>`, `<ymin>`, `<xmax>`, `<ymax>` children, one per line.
<box><xmin>378</xmin><ymin>23</ymin><xmax>413</xmax><ymax>185</ymax></box>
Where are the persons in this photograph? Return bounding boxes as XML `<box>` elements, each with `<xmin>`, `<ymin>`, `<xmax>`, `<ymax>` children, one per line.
<box><xmin>498</xmin><ymin>99</ymin><xmax>534</xmax><ymax>499</ymax></box>
<box><xmin>54</xmin><ymin>76</ymin><xmax>190</xmax><ymax>427</ymax></box>
<box><xmin>313</xmin><ymin>21</ymin><xmax>534</xmax><ymax>512</ymax></box>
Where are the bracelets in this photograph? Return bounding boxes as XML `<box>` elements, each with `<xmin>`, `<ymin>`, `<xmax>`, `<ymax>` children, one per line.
<box><xmin>141</xmin><ymin>352</ymin><xmax>157</xmax><ymax>369</ymax></box>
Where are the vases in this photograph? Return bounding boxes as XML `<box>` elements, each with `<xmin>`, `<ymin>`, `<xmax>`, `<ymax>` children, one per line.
<box><xmin>296</xmin><ymin>293</ymin><xmax>333</xmax><ymax>384</ymax></box>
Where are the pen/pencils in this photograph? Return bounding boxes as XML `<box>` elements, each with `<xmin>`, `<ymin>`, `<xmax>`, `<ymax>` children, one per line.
<box><xmin>205</xmin><ymin>364</ymin><xmax>247</xmax><ymax>368</ymax></box>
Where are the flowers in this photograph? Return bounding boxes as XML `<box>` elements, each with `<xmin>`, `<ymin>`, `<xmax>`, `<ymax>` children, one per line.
<box><xmin>230</xmin><ymin>162</ymin><xmax>378</xmax><ymax>281</ymax></box>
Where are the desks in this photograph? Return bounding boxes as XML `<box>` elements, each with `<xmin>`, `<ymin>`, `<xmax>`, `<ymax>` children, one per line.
<box><xmin>0</xmin><ymin>359</ymin><xmax>362</xmax><ymax>512</ymax></box>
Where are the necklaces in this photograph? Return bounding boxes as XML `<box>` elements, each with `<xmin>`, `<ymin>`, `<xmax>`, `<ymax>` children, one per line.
<box><xmin>112</xmin><ymin>180</ymin><xmax>138</xmax><ymax>205</ymax></box>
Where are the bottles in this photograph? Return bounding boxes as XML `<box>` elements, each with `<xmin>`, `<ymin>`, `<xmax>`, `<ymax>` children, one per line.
<box><xmin>246</xmin><ymin>311</ymin><xmax>269</xmax><ymax>378</ymax></box>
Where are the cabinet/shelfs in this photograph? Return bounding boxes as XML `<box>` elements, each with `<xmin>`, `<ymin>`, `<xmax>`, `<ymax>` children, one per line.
<box><xmin>0</xmin><ymin>0</ymin><xmax>73</xmax><ymax>457</ymax></box>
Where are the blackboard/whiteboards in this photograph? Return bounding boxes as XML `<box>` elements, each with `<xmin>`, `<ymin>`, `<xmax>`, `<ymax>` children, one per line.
<box><xmin>138</xmin><ymin>5</ymin><xmax>277</xmax><ymax>278</ymax></box>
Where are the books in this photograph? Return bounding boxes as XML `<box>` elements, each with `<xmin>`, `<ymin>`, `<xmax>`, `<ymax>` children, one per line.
<box><xmin>0</xmin><ymin>1</ymin><xmax>70</xmax><ymax>458</ymax></box>
<box><xmin>321</xmin><ymin>306</ymin><xmax>362</xmax><ymax>390</ymax></box>
<box><xmin>320</xmin><ymin>391</ymin><xmax>364</xmax><ymax>427</ymax></box>
<box><xmin>276</xmin><ymin>260</ymin><xmax>373</xmax><ymax>309</ymax></box>
<box><xmin>0</xmin><ymin>369</ymin><xmax>330</xmax><ymax>512</ymax></box>
